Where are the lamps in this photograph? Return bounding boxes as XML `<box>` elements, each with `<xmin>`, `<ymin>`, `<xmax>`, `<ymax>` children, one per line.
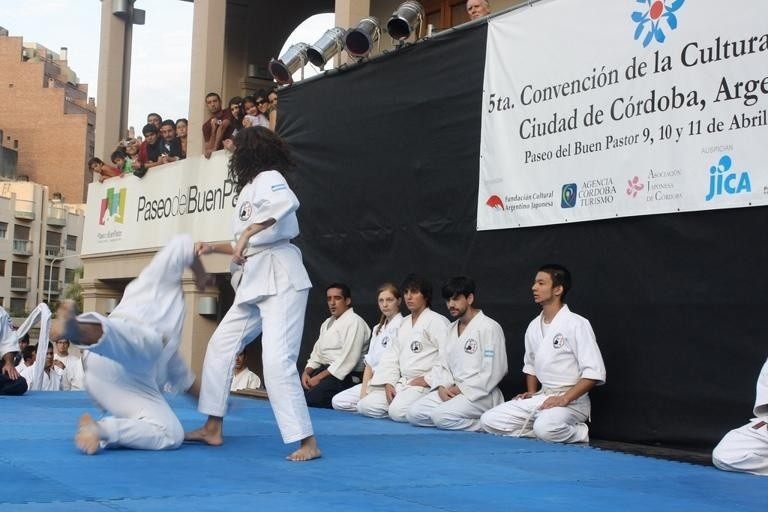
<box><xmin>262</xmin><ymin>1</ymin><xmax>428</xmax><ymax>88</ymax></box>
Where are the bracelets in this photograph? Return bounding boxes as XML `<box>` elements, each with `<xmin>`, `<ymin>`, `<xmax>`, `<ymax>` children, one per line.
<box><xmin>210</xmin><ymin>243</ymin><xmax>215</xmax><ymax>252</ymax></box>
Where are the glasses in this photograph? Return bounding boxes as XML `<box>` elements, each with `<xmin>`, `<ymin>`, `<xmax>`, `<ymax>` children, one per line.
<box><xmin>255</xmin><ymin>98</ymin><xmax>267</xmax><ymax>106</ymax></box>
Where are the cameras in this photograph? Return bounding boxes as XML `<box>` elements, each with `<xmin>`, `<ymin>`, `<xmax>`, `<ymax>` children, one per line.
<box><xmin>123</xmin><ymin>141</ymin><xmax>131</xmax><ymax>146</ymax></box>
<box><xmin>214</xmin><ymin>120</ymin><xmax>223</xmax><ymax>126</ymax></box>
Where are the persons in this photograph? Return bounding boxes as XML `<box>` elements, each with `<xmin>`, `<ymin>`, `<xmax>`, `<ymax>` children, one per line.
<box><xmin>711</xmin><ymin>358</ymin><xmax>767</xmax><ymax>478</ymax></box>
<box><xmin>331</xmin><ymin>281</ymin><xmax>404</xmax><ymax>418</ymax></box>
<box><xmin>466</xmin><ymin>0</ymin><xmax>490</xmax><ymax>21</ymax></box>
<box><xmin>0</xmin><ymin>302</ymin><xmax>110</xmax><ymax>396</ymax></box>
<box><xmin>182</xmin><ymin>126</ymin><xmax>323</xmax><ymax>460</ymax></box>
<box><xmin>356</xmin><ymin>274</ymin><xmax>452</xmax><ymax>421</ymax></box>
<box><xmin>85</xmin><ymin>85</ymin><xmax>277</xmax><ymax>183</ymax></box>
<box><xmin>480</xmin><ymin>264</ymin><xmax>608</xmax><ymax>443</ymax></box>
<box><xmin>406</xmin><ymin>275</ymin><xmax>510</xmax><ymax>432</ymax></box>
<box><xmin>229</xmin><ymin>348</ymin><xmax>262</xmax><ymax>391</ymax></box>
<box><xmin>50</xmin><ymin>233</ymin><xmax>242</xmax><ymax>454</ymax></box>
<box><xmin>300</xmin><ymin>283</ymin><xmax>371</xmax><ymax>408</ymax></box>
